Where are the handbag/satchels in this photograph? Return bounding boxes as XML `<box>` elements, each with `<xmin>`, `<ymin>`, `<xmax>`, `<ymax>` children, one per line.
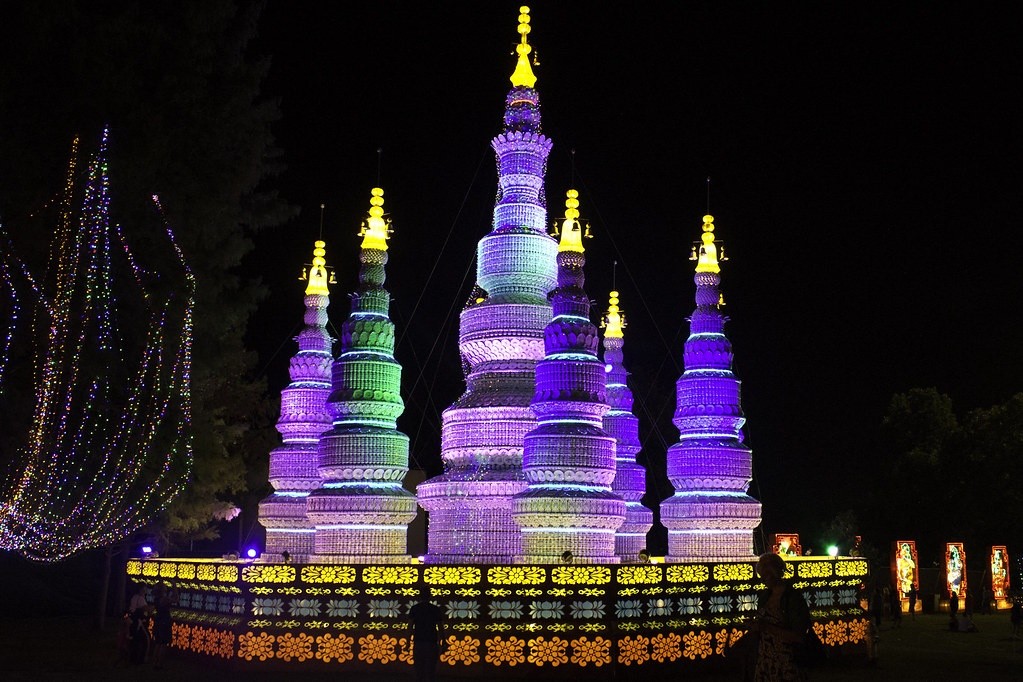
<box><xmin>781</xmin><ymin>589</ymin><xmax>831</xmax><ymax>673</ymax></box>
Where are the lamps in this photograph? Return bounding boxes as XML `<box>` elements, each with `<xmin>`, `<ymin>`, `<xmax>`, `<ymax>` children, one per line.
<box><xmin>418</xmin><ymin>555</ymin><xmax>425</xmax><ymax>565</ymax></box>
<box><xmin>282</xmin><ymin>552</ymin><xmax>293</xmax><ymax>565</ymax></box>
<box><xmin>561</xmin><ymin>551</ymin><xmax>575</xmax><ymax>565</ymax></box>
<box><xmin>228</xmin><ymin>550</ymin><xmax>240</xmax><ymax>563</ymax></box>
<box><xmin>638</xmin><ymin>549</ymin><xmax>651</xmax><ymax>564</ymax></box>
<box><xmin>248</xmin><ymin>549</ymin><xmax>257</xmax><ymax>560</ymax></box>
<box><xmin>141</xmin><ymin>544</ymin><xmax>158</xmax><ymax>560</ymax></box>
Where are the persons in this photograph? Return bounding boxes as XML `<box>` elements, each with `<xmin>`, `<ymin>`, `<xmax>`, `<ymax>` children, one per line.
<box><xmin>872</xmin><ymin>580</ymin><xmax>1023</xmax><ymax>641</ymax></box>
<box><xmin>115</xmin><ymin>579</ymin><xmax>179</xmax><ymax>670</ymax></box>
<box><xmin>403</xmin><ymin>584</ymin><xmax>445</xmax><ymax>682</ymax></box>
<box><xmin>750</xmin><ymin>554</ymin><xmax>811</xmax><ymax>682</ymax></box>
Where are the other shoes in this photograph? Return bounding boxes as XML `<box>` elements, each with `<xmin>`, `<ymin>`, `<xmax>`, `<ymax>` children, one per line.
<box><xmin>1009</xmin><ymin>635</ymin><xmax>1014</xmax><ymax>640</ymax></box>
<box><xmin>1014</xmin><ymin>634</ymin><xmax>1020</xmax><ymax>640</ymax></box>
